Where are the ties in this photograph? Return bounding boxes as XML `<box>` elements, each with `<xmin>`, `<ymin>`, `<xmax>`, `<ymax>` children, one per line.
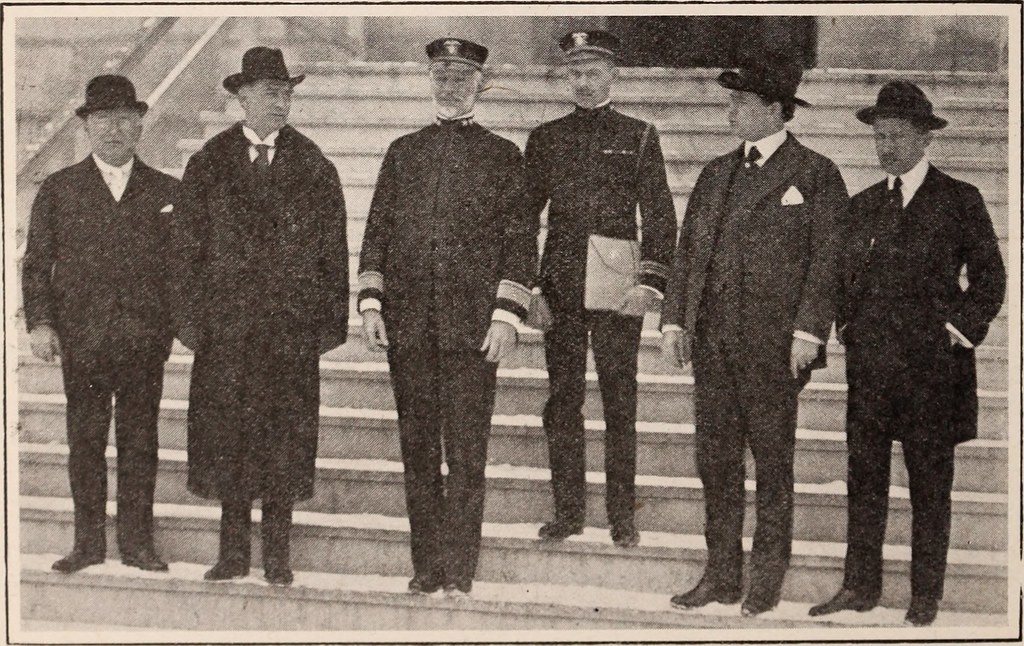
<box><xmin>709</xmin><ymin>146</ymin><xmax>762</xmax><ymax>294</ymax></box>
<box><xmin>879</xmin><ymin>177</ymin><xmax>903</xmax><ymax>231</ymax></box>
<box><xmin>252</xmin><ymin>144</ymin><xmax>270</xmax><ymax>210</ymax></box>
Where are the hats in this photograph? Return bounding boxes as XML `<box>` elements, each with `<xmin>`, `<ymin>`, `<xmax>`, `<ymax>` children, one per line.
<box><xmin>559</xmin><ymin>30</ymin><xmax>628</xmax><ymax>61</ymax></box>
<box><xmin>223</xmin><ymin>47</ymin><xmax>306</xmax><ymax>95</ymax></box>
<box><xmin>717</xmin><ymin>52</ymin><xmax>813</xmax><ymax>108</ymax></box>
<box><xmin>854</xmin><ymin>81</ymin><xmax>948</xmax><ymax>131</ymax></box>
<box><xmin>425</xmin><ymin>38</ymin><xmax>489</xmax><ymax>69</ymax></box>
<box><xmin>76</xmin><ymin>75</ymin><xmax>149</xmax><ymax>117</ymax></box>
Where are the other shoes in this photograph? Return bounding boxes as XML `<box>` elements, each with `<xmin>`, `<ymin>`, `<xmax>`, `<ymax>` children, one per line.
<box><xmin>265</xmin><ymin>563</ymin><xmax>294</xmax><ymax>584</ymax></box>
<box><xmin>52</xmin><ymin>551</ymin><xmax>106</xmax><ymax>575</ymax></box>
<box><xmin>539</xmin><ymin>518</ymin><xmax>583</xmax><ymax>538</ymax></box>
<box><xmin>611</xmin><ymin>524</ymin><xmax>642</xmax><ymax>549</ymax></box>
<box><xmin>671</xmin><ymin>578</ymin><xmax>741</xmax><ymax>611</ymax></box>
<box><xmin>810</xmin><ymin>587</ymin><xmax>883</xmax><ymax>616</ymax></box>
<box><xmin>409</xmin><ymin>575</ymin><xmax>443</xmax><ymax>594</ymax></box>
<box><xmin>204</xmin><ymin>561</ymin><xmax>250</xmax><ymax>581</ymax></box>
<box><xmin>904</xmin><ymin>596</ymin><xmax>939</xmax><ymax>626</ymax></box>
<box><xmin>738</xmin><ymin>597</ymin><xmax>780</xmax><ymax>617</ymax></box>
<box><xmin>443</xmin><ymin>579</ymin><xmax>471</xmax><ymax>595</ymax></box>
<box><xmin>122</xmin><ymin>548</ymin><xmax>168</xmax><ymax>572</ymax></box>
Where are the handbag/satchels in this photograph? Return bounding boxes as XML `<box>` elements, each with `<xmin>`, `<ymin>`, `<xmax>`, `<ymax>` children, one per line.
<box><xmin>582</xmin><ymin>237</ymin><xmax>643</xmax><ymax>311</ymax></box>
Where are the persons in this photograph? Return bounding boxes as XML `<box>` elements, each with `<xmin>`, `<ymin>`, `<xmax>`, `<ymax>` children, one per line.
<box><xmin>807</xmin><ymin>78</ymin><xmax>1006</xmax><ymax>627</ymax></box>
<box><xmin>357</xmin><ymin>37</ymin><xmax>540</xmax><ymax>593</ymax></box>
<box><xmin>169</xmin><ymin>46</ymin><xmax>350</xmax><ymax>585</ymax></box>
<box><xmin>524</xmin><ymin>30</ymin><xmax>677</xmax><ymax>549</ymax></box>
<box><xmin>659</xmin><ymin>50</ymin><xmax>851</xmax><ymax>616</ymax></box>
<box><xmin>22</xmin><ymin>74</ymin><xmax>182</xmax><ymax>573</ymax></box>
